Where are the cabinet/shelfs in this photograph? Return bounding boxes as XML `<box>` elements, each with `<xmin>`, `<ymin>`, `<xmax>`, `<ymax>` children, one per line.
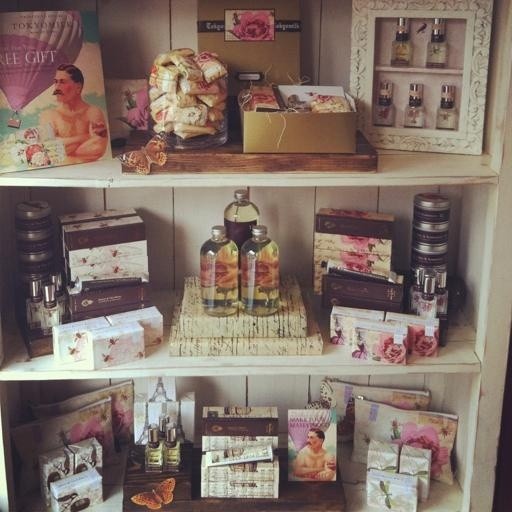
<box><xmin>0</xmin><ymin>0</ymin><xmax>512</xmax><ymax>512</ymax></box>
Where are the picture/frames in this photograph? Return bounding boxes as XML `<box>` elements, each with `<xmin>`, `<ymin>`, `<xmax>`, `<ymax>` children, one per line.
<box><xmin>349</xmin><ymin>0</ymin><xmax>493</xmax><ymax>156</ymax></box>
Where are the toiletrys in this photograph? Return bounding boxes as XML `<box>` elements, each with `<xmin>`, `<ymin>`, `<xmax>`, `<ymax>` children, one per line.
<box><xmin>406</xmin><ymin>266</ymin><xmax>449</xmax><ymax>318</ymax></box>
<box><xmin>372</xmin><ymin>13</ymin><xmax>459</xmax><ymax>130</ymax></box>
<box><xmin>143</xmin><ymin>414</ymin><xmax>185</xmax><ymax>472</ymax></box>
<box><xmin>26</xmin><ymin>269</ymin><xmax>71</xmax><ymax>335</ymax></box>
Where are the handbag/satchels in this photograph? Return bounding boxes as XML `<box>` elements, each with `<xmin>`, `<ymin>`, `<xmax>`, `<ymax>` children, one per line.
<box><xmin>351</xmin><ymin>396</ymin><xmax>458</xmax><ymax>486</ymax></box>
<box><xmin>322</xmin><ymin>380</ymin><xmax>430</xmax><ymax>445</ymax></box>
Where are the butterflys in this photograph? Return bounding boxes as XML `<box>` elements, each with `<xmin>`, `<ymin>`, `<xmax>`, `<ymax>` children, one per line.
<box><xmin>129</xmin><ymin>477</ymin><xmax>176</xmax><ymax>511</ymax></box>
<box><xmin>115</xmin><ymin>132</ymin><xmax>169</xmax><ymax>175</ymax></box>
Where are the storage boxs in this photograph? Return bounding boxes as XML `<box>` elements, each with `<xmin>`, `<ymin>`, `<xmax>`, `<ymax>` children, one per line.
<box><xmin>239</xmin><ymin>85</ymin><xmax>357</xmax><ymax>154</ymax></box>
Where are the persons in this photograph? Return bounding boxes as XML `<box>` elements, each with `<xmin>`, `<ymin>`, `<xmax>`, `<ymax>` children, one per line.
<box><xmin>36</xmin><ymin>64</ymin><xmax>109</xmax><ymax>166</ymax></box>
<box><xmin>293</xmin><ymin>428</ymin><xmax>339</xmax><ymax>487</ymax></box>
